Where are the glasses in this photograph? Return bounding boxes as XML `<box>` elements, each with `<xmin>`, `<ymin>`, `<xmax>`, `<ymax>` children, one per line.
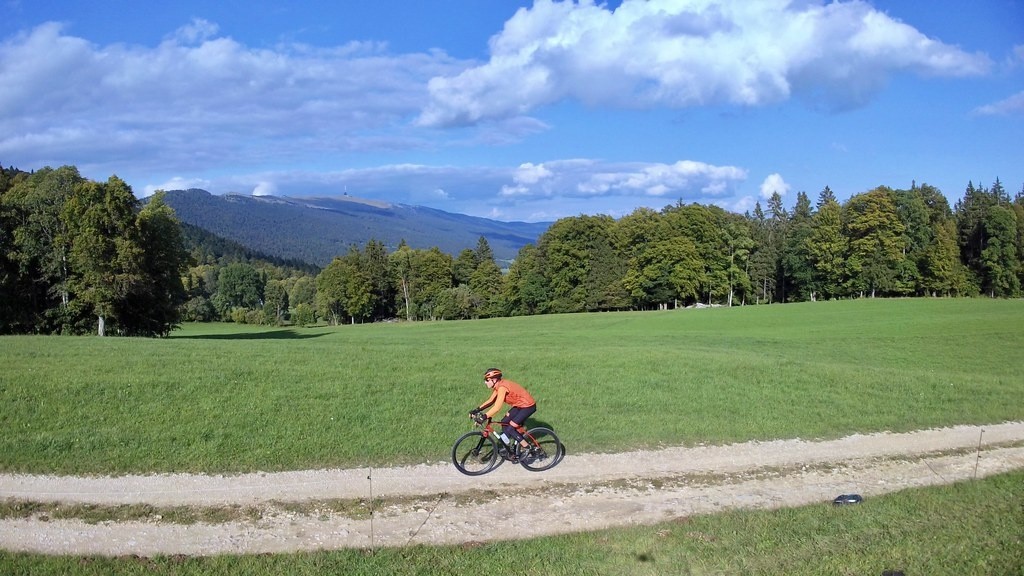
<box><xmin>485</xmin><ymin>377</ymin><xmax>493</xmax><ymax>382</ymax></box>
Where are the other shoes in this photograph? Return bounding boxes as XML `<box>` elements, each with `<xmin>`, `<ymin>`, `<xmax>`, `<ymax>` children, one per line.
<box><xmin>520</xmin><ymin>445</ymin><xmax>534</xmax><ymax>461</ymax></box>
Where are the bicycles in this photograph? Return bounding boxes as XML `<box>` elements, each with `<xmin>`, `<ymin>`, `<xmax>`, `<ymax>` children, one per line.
<box><xmin>451</xmin><ymin>410</ymin><xmax>561</xmax><ymax>477</ymax></box>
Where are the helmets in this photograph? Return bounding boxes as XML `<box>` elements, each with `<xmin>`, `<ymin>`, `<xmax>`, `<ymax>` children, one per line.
<box><xmin>484</xmin><ymin>368</ymin><xmax>502</xmax><ymax>379</ymax></box>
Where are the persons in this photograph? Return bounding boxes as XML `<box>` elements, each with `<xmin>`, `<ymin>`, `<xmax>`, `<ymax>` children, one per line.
<box><xmin>469</xmin><ymin>368</ymin><xmax>536</xmax><ymax>462</ymax></box>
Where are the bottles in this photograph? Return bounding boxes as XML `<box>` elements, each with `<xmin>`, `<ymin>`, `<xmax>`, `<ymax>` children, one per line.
<box><xmin>500</xmin><ymin>432</ymin><xmax>510</xmax><ymax>445</ymax></box>
